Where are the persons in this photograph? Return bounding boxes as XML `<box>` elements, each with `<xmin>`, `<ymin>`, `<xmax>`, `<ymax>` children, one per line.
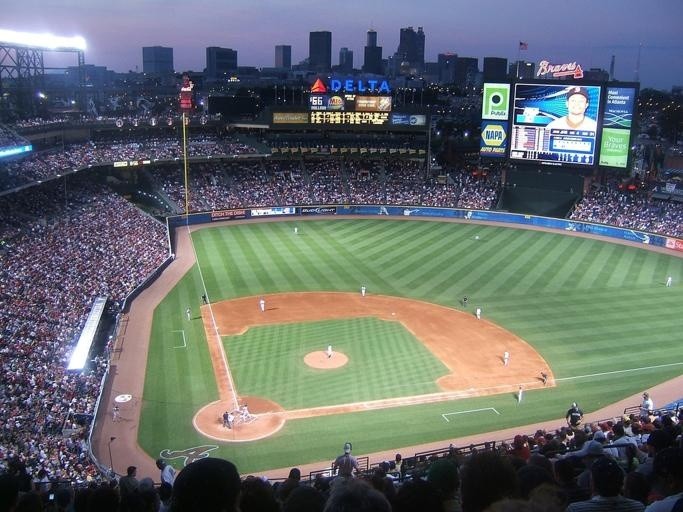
<box><xmin>540</xmin><ymin>371</ymin><xmax>548</xmax><ymax>388</ymax></box>
<box><xmin>475</xmin><ymin>306</ymin><xmax>481</xmax><ymax>320</ymax></box>
<box><xmin>0</xmin><ymin>109</ymin><xmax>173</xmax><ymax>512</ymax></box>
<box><xmin>361</xmin><ymin>285</ymin><xmax>366</xmax><ymax>297</ymax></box>
<box><xmin>175</xmin><ymin>133</ymin><xmax>682</xmax><ymax>239</ymax></box>
<box><xmin>201</xmin><ymin>292</ymin><xmax>207</xmax><ymax>306</ymax></box>
<box><xmin>543</xmin><ymin>87</ymin><xmax>597</xmax><ymax>131</ymax></box>
<box><xmin>172</xmin><ymin>387</ymin><xmax>683</xmax><ymax>512</ymax></box>
<box><xmin>186</xmin><ymin>304</ymin><xmax>192</xmax><ymax>324</ymax></box>
<box><xmin>258</xmin><ymin>298</ymin><xmax>264</xmax><ymax>314</ymax></box>
<box><xmin>503</xmin><ymin>350</ymin><xmax>509</xmax><ymax>368</ymax></box>
<box><xmin>462</xmin><ymin>294</ymin><xmax>468</xmax><ymax>308</ymax></box>
<box><xmin>665</xmin><ymin>275</ymin><xmax>672</xmax><ymax>288</ymax></box>
<box><xmin>327</xmin><ymin>343</ymin><xmax>332</xmax><ymax>358</ymax></box>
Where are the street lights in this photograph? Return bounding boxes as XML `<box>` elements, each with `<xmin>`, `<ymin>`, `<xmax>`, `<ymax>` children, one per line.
<box><xmin>105</xmin><ymin>435</ymin><xmax>115</xmax><ymax>472</ymax></box>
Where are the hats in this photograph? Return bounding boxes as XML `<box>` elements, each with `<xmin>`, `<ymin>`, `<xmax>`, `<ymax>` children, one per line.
<box><xmin>567</xmin><ymin>86</ymin><xmax>590</xmax><ymax>99</ymax></box>
<box><xmin>156</xmin><ymin>459</ymin><xmax>167</xmax><ymax>467</ymax></box>
<box><xmin>344</xmin><ymin>443</ymin><xmax>353</xmax><ymax>454</ymax></box>
<box><xmin>513</xmin><ymin>391</ymin><xmax>650</xmax><ymax>443</ymax></box>
